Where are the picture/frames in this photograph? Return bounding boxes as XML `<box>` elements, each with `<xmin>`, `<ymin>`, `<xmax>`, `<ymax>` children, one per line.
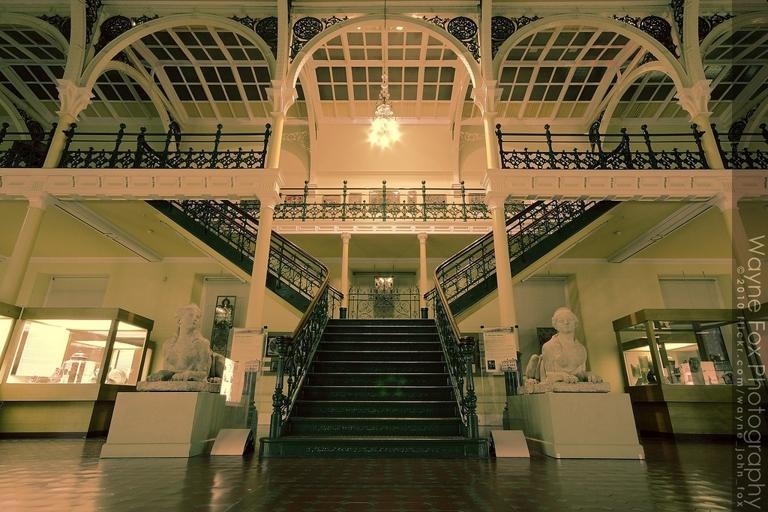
<box><xmin>485</xmin><ymin>359</ymin><xmax>497</xmax><ymax>372</ymax></box>
<box><xmin>266</xmin><ymin>336</ymin><xmax>279</xmax><ymax>357</ymax></box>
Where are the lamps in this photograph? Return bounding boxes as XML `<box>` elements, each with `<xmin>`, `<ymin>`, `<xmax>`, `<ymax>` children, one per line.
<box><xmin>368</xmin><ymin>0</ymin><xmax>399</xmax><ymax>146</ymax></box>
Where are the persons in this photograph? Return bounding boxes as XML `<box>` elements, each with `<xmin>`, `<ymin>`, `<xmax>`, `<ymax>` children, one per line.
<box><xmin>145</xmin><ymin>303</ymin><xmax>240</xmax><ymax>380</ymax></box>
<box><xmin>522</xmin><ymin>307</ymin><xmax>603</xmax><ymax>383</ymax></box>
